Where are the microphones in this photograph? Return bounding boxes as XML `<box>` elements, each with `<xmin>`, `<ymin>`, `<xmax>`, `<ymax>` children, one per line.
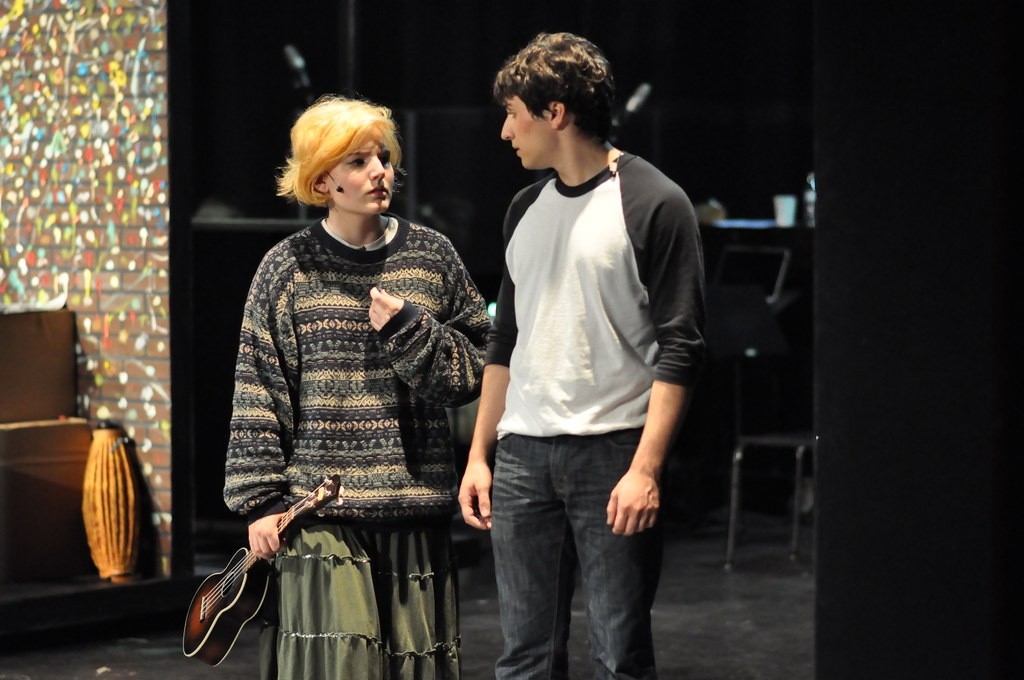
<box><xmin>325</xmin><ymin>172</ymin><xmax>343</xmax><ymax>192</ymax></box>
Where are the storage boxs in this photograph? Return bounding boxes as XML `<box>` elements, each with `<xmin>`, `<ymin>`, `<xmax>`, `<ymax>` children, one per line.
<box><xmin>0</xmin><ymin>309</ymin><xmax>93</xmax><ymax>581</ymax></box>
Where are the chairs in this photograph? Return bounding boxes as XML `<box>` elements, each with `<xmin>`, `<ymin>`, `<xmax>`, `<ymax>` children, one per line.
<box><xmin>723</xmin><ymin>347</ymin><xmax>815</xmax><ymax>572</ymax></box>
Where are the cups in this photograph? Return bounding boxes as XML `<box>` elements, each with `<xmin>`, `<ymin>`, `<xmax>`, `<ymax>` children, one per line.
<box><xmin>772</xmin><ymin>194</ymin><xmax>797</xmax><ymax>228</ymax></box>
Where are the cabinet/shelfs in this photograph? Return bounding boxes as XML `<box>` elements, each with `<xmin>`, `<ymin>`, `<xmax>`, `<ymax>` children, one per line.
<box><xmin>189</xmin><ymin>216</ymin><xmax>315</xmax><ymax>548</ymax></box>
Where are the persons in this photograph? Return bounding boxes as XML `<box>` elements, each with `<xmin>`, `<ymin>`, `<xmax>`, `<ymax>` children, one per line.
<box><xmin>456</xmin><ymin>33</ymin><xmax>706</xmax><ymax>680</ymax></box>
<box><xmin>222</xmin><ymin>95</ymin><xmax>491</xmax><ymax>680</ymax></box>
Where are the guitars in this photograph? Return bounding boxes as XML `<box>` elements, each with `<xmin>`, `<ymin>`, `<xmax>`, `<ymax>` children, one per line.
<box><xmin>180</xmin><ymin>471</ymin><xmax>346</xmax><ymax>668</ymax></box>
